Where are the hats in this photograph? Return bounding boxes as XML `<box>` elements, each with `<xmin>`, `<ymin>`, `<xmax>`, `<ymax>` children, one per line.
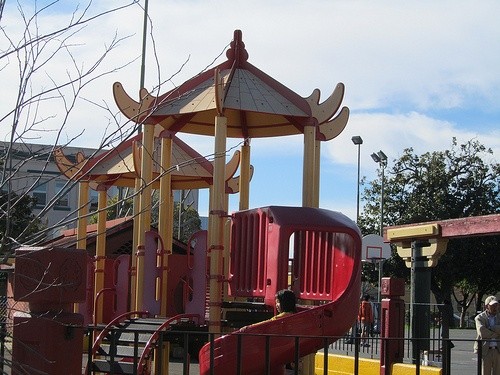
<box><xmin>485</xmin><ymin>296</ymin><xmax>498</xmax><ymax>306</ymax></box>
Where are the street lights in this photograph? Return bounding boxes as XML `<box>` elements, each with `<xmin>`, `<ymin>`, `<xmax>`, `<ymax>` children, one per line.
<box><xmin>351</xmin><ymin>135</ymin><xmax>363</xmax><ymax>224</ymax></box>
<box><xmin>369</xmin><ymin>149</ymin><xmax>388</xmax><ymax>303</ymax></box>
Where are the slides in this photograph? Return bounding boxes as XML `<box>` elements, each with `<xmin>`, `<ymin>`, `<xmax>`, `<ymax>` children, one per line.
<box><xmin>200</xmin><ymin>206</ymin><xmax>362</xmax><ymax>375</ymax></box>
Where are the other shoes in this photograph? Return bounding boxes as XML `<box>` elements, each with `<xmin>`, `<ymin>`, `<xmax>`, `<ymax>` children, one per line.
<box><xmin>361</xmin><ymin>343</ymin><xmax>371</xmax><ymax>347</ymax></box>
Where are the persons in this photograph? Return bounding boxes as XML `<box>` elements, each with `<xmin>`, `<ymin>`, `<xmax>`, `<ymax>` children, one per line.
<box><xmin>473</xmin><ymin>296</ymin><xmax>500</xmax><ymax>375</ymax></box>
<box><xmin>268</xmin><ymin>289</ymin><xmax>297</xmax><ymax>318</ymax></box>
<box><xmin>344</xmin><ymin>293</ymin><xmax>378</xmax><ymax>347</ymax></box>
<box><xmin>430</xmin><ymin>311</ymin><xmax>443</xmax><ymax>328</ymax></box>
